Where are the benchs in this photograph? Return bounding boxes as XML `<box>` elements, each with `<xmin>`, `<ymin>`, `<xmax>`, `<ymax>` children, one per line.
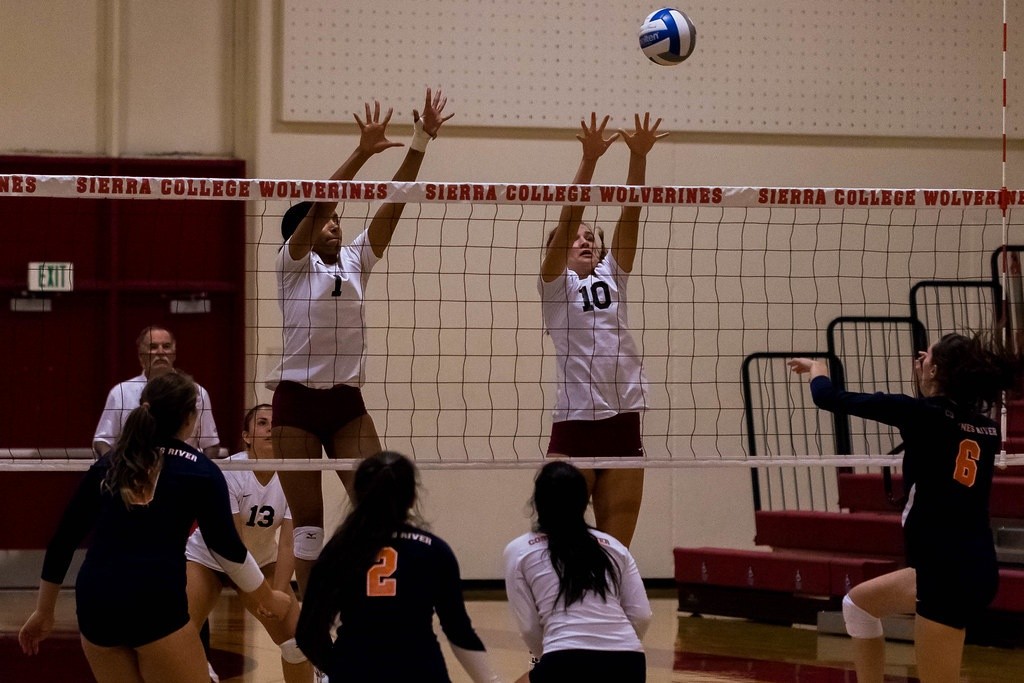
<box><xmin>670</xmin><ymin>393</ymin><xmax>1024</xmax><ymax>630</ymax></box>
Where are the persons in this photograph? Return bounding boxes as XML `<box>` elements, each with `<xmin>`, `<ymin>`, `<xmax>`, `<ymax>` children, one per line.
<box><xmin>787</xmin><ymin>330</ymin><xmax>1021</xmax><ymax>681</ymax></box>
<box><xmin>17</xmin><ymin>371</ymin><xmax>291</xmax><ymax>682</ymax></box>
<box><xmin>539</xmin><ymin>112</ymin><xmax>671</xmax><ymax>554</ymax></box>
<box><xmin>297</xmin><ymin>450</ymin><xmax>499</xmax><ymax>683</ymax></box>
<box><xmin>265</xmin><ymin>86</ymin><xmax>455</xmax><ymax>683</ymax></box>
<box><xmin>92</xmin><ymin>324</ymin><xmax>222</xmax><ymax>464</ymax></box>
<box><xmin>502</xmin><ymin>462</ymin><xmax>651</xmax><ymax>683</ymax></box>
<box><xmin>195</xmin><ymin>402</ymin><xmax>316</xmax><ymax>682</ymax></box>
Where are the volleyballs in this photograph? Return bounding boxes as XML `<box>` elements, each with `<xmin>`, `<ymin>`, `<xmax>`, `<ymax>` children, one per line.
<box><xmin>640</xmin><ymin>7</ymin><xmax>697</xmax><ymax>67</ymax></box>
